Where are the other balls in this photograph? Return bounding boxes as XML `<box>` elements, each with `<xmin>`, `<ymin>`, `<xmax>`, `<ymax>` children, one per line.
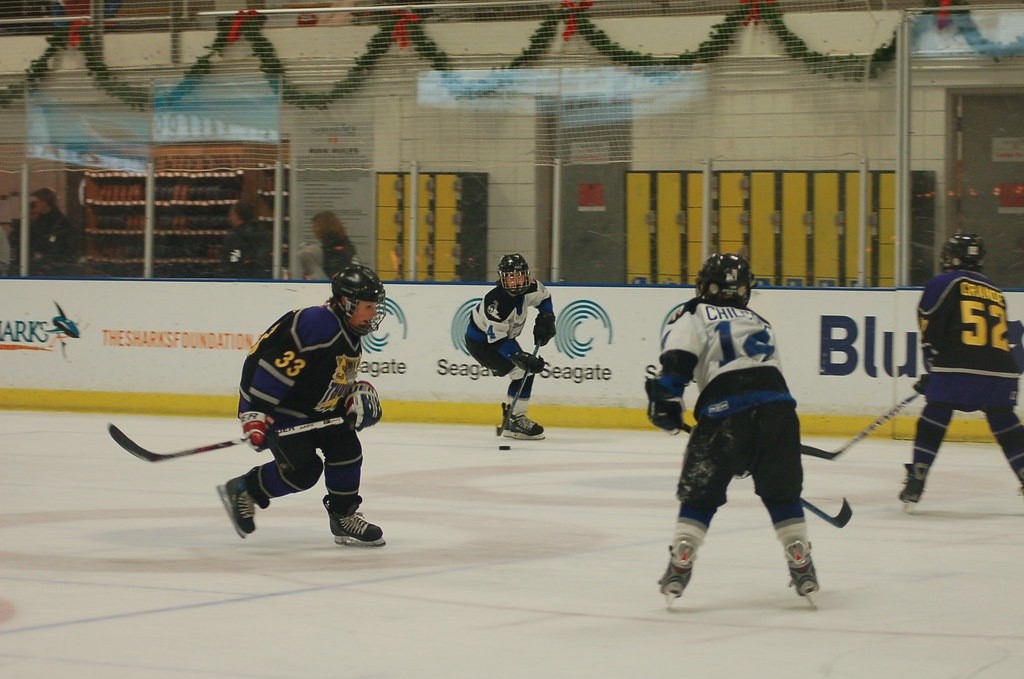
<box><xmin>499</xmin><ymin>445</ymin><xmax>511</xmax><ymax>450</ymax></box>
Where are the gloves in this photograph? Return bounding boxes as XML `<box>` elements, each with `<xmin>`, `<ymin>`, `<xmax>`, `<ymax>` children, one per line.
<box><xmin>511</xmin><ymin>350</ymin><xmax>545</xmax><ymax>373</ymax></box>
<box><xmin>533</xmin><ymin>311</ymin><xmax>556</xmax><ymax>346</ymax></box>
<box><xmin>646</xmin><ymin>375</ymin><xmax>687</xmax><ymax>432</ymax></box>
<box><xmin>240</xmin><ymin>401</ymin><xmax>278</xmax><ymax>452</ymax></box>
<box><xmin>345</xmin><ymin>380</ymin><xmax>383</xmax><ymax>432</ymax></box>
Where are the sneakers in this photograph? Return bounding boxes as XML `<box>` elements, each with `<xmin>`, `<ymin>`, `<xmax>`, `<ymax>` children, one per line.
<box><xmin>784</xmin><ymin>568</ymin><xmax>820</xmax><ymax>610</ymax></box>
<box><xmin>898</xmin><ymin>462</ymin><xmax>930</xmax><ymax>515</ymax></box>
<box><xmin>502</xmin><ymin>412</ymin><xmax>545</xmax><ymax>440</ymax></box>
<box><xmin>329</xmin><ymin>511</ymin><xmax>388</xmax><ymax>548</ymax></box>
<box><xmin>658</xmin><ymin>561</ymin><xmax>697</xmax><ymax>608</ymax></box>
<box><xmin>217</xmin><ymin>472</ymin><xmax>256</xmax><ymax>541</ymax></box>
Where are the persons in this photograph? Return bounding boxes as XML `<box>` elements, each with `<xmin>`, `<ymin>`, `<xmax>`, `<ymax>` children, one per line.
<box><xmin>223</xmin><ymin>265</ymin><xmax>386</xmax><ymax>541</ymax></box>
<box><xmin>219</xmin><ymin>201</ymin><xmax>287</xmax><ymax>280</ymax></box>
<box><xmin>29</xmin><ymin>188</ymin><xmax>79</xmax><ymax>278</ymax></box>
<box><xmin>646</xmin><ymin>254</ymin><xmax>820</xmax><ymax>598</ymax></box>
<box><xmin>465</xmin><ymin>252</ymin><xmax>555</xmax><ymax>435</ymax></box>
<box><xmin>898</xmin><ymin>230</ymin><xmax>1024</xmax><ymax>502</ymax></box>
<box><xmin>294</xmin><ymin>209</ymin><xmax>361</xmax><ymax>282</ymax></box>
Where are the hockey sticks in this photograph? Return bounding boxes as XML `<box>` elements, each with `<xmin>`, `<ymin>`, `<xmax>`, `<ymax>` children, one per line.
<box><xmin>679</xmin><ymin>416</ymin><xmax>854</xmax><ymax>529</ymax></box>
<box><xmin>495</xmin><ymin>337</ymin><xmax>542</xmax><ymax>437</ymax></box>
<box><xmin>107</xmin><ymin>416</ymin><xmax>346</xmax><ymax>463</ymax></box>
<box><xmin>797</xmin><ymin>390</ymin><xmax>921</xmax><ymax>461</ymax></box>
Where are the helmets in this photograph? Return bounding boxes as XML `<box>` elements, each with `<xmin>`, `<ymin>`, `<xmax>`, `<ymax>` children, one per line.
<box><xmin>695</xmin><ymin>252</ymin><xmax>755</xmax><ymax>310</ymax></box>
<box><xmin>941</xmin><ymin>232</ymin><xmax>985</xmax><ymax>272</ymax></box>
<box><xmin>330</xmin><ymin>263</ymin><xmax>386</xmax><ymax>336</ymax></box>
<box><xmin>497</xmin><ymin>253</ymin><xmax>530</xmax><ymax>296</ymax></box>
